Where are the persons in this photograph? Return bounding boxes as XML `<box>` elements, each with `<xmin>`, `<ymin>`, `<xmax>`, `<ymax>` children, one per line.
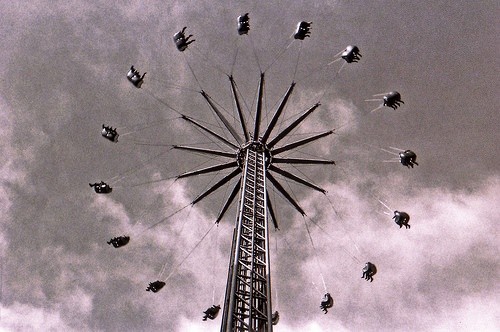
<box><xmin>89</xmin><ymin>13</ymin><xmax>419</xmax><ymax>324</ymax></box>
